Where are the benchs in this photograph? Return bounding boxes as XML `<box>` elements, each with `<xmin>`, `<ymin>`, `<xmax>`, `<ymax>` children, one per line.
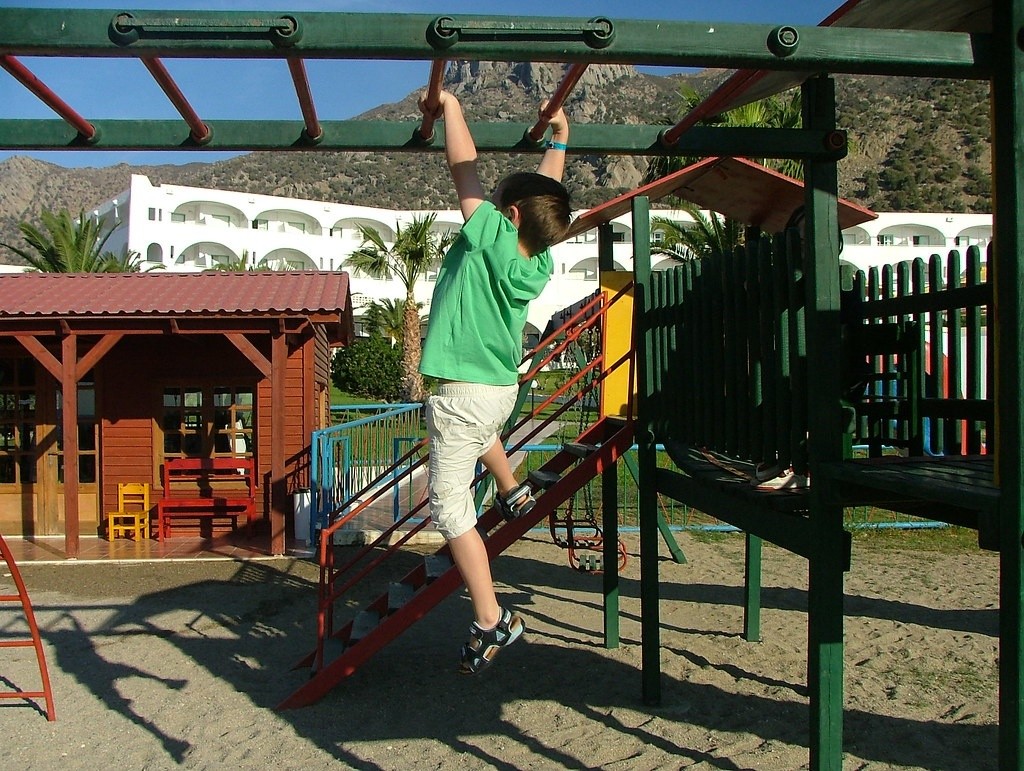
<box><xmin>157</xmin><ymin>458</ymin><xmax>256</xmax><ymax>540</ymax></box>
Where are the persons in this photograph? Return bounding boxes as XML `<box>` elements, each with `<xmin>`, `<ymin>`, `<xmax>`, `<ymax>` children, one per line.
<box><xmin>747</xmin><ymin>202</ymin><xmax>872</xmax><ymax>498</ymax></box>
<box><xmin>416</xmin><ymin>88</ymin><xmax>572</xmax><ymax>678</ymax></box>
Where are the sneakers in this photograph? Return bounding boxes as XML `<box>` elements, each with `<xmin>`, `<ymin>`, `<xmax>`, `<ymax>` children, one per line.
<box><xmin>756</xmin><ymin>464</ymin><xmax>787</xmax><ymax>481</ymax></box>
<box><xmin>756</xmin><ymin>467</ymin><xmax>812</xmax><ymax>496</ymax></box>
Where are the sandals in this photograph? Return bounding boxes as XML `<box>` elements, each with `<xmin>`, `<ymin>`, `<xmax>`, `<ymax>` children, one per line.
<box><xmin>494</xmin><ymin>481</ymin><xmax>536</xmax><ymax>519</ymax></box>
<box><xmin>456</xmin><ymin>606</ymin><xmax>525</xmax><ymax>678</ymax></box>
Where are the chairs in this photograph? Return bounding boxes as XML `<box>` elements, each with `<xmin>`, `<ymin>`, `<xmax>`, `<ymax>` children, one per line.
<box><xmin>109</xmin><ymin>483</ymin><xmax>149</xmax><ymax>542</ymax></box>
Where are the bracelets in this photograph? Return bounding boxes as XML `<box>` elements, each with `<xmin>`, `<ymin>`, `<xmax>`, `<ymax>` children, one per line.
<box><xmin>545</xmin><ymin>140</ymin><xmax>568</xmax><ymax>151</ymax></box>
